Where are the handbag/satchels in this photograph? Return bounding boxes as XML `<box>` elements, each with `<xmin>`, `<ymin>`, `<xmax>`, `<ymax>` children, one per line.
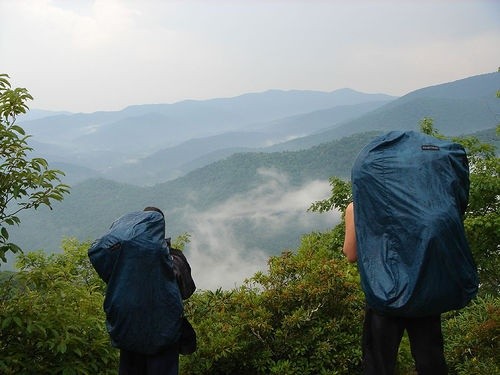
<box><xmin>182</xmin><ymin>314</ymin><xmax>199</xmax><ymax>357</ymax></box>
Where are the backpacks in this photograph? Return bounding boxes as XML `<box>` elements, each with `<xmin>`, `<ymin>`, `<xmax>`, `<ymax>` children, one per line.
<box><xmin>84</xmin><ymin>210</ymin><xmax>182</xmax><ymax>355</ymax></box>
<box><xmin>350</xmin><ymin>131</ymin><xmax>482</xmax><ymax>313</ymax></box>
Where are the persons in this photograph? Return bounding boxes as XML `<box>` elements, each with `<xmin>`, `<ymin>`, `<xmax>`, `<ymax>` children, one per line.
<box><xmin>118</xmin><ymin>206</ymin><xmax>197</xmax><ymax>375</ymax></box>
<box><xmin>341</xmin><ymin>202</ymin><xmax>449</xmax><ymax>375</ymax></box>
